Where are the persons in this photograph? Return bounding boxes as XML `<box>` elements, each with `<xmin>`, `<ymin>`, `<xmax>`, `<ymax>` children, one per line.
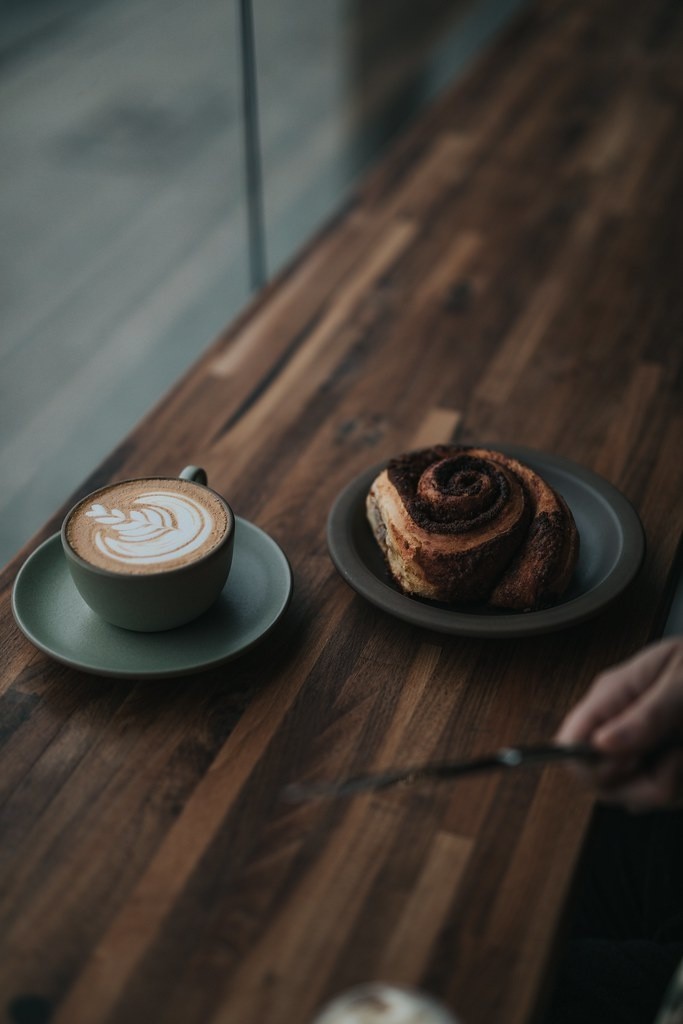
<box><xmin>550</xmin><ymin>635</ymin><xmax>682</xmax><ymax>812</ymax></box>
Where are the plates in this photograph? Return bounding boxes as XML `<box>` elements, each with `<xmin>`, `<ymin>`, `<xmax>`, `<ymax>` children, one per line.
<box><xmin>10</xmin><ymin>513</ymin><xmax>291</xmax><ymax>675</ymax></box>
<box><xmin>324</xmin><ymin>441</ymin><xmax>641</xmax><ymax>634</ymax></box>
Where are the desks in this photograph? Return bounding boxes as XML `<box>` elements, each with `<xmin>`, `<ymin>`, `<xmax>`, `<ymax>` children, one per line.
<box><xmin>0</xmin><ymin>0</ymin><xmax>683</xmax><ymax>1023</ymax></box>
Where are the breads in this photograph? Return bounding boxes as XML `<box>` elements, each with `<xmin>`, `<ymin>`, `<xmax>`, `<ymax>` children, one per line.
<box><xmin>365</xmin><ymin>443</ymin><xmax>580</xmax><ymax>615</ymax></box>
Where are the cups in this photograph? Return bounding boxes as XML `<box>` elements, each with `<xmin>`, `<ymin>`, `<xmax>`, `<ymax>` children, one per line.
<box><xmin>60</xmin><ymin>465</ymin><xmax>235</xmax><ymax>634</ymax></box>
